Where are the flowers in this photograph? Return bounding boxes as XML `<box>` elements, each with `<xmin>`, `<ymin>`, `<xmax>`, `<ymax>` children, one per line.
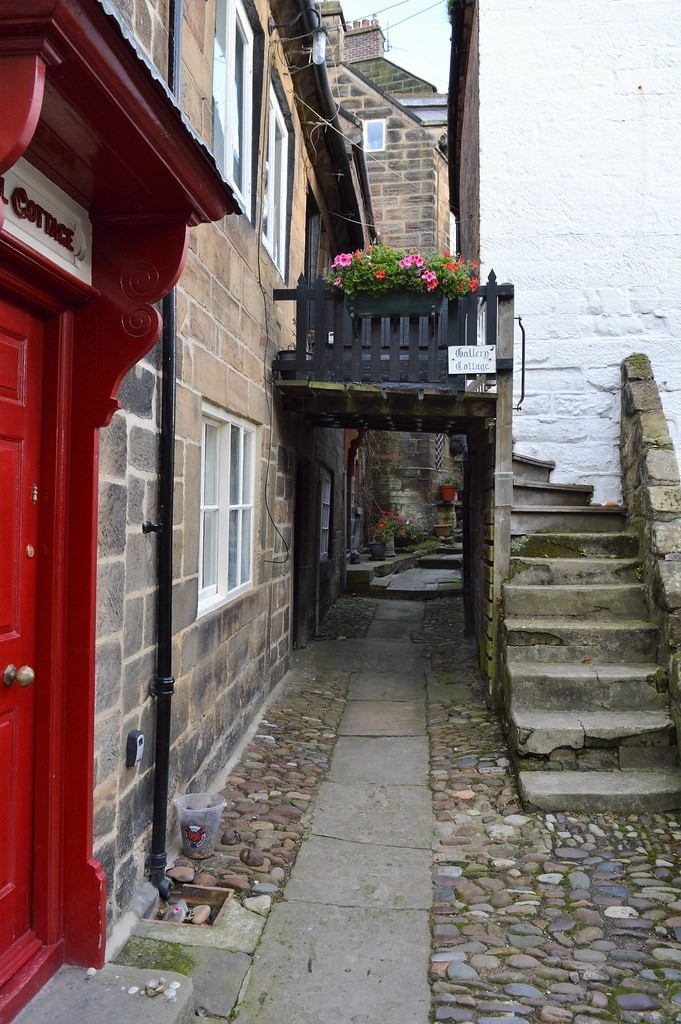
<box><xmin>369</xmin><ymin>508</ymin><xmax>416</xmax><ymax>547</ymax></box>
<box><xmin>321</xmin><ymin>243</ymin><xmax>479</xmax><ymax>301</ymax></box>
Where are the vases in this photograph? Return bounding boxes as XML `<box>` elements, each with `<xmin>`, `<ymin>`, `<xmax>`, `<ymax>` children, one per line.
<box><xmin>362</xmin><ymin>19</ymin><xmax>370</xmax><ymax>26</ymax></box>
<box><xmin>371</xmin><ymin>19</ymin><xmax>379</xmax><ymax>25</ymax></box>
<box><xmin>378</xmin><ymin>534</ymin><xmax>397</xmax><ymax>556</ymax></box>
<box><xmin>353</xmin><ymin>20</ymin><xmax>360</xmax><ymax>28</ymax></box>
<box><xmin>435</xmin><ymin>524</ymin><xmax>452</xmax><ymax>537</ymax></box>
<box><xmin>344</xmin><ymin>288</ymin><xmax>445</xmax><ymax>317</ymax></box>
<box><xmin>368</xmin><ymin>542</ymin><xmax>386</xmax><ymax>557</ymax></box>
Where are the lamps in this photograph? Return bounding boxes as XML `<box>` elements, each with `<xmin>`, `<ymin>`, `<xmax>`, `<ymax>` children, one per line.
<box><xmin>268</xmin><ymin>8</ymin><xmax>328</xmax><ymax>65</ymax></box>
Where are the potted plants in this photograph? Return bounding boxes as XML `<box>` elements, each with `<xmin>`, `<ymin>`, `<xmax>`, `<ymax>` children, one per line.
<box><xmin>439</xmin><ymin>477</ymin><xmax>457</xmax><ymax>500</ymax></box>
